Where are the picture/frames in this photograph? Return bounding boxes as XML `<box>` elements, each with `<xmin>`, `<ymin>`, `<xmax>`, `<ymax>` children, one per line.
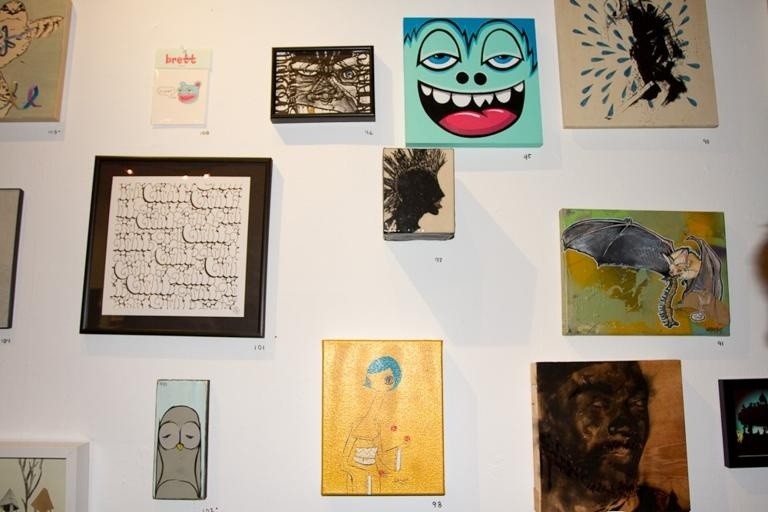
<box><xmin>0</xmin><ymin>441</ymin><xmax>90</xmax><ymax>512</ymax></box>
<box><xmin>270</xmin><ymin>45</ymin><xmax>376</xmax><ymax>124</ymax></box>
<box><xmin>79</xmin><ymin>155</ymin><xmax>273</xmax><ymax>338</ymax></box>
<box><xmin>718</xmin><ymin>378</ymin><xmax>768</xmax><ymax>467</ymax></box>
